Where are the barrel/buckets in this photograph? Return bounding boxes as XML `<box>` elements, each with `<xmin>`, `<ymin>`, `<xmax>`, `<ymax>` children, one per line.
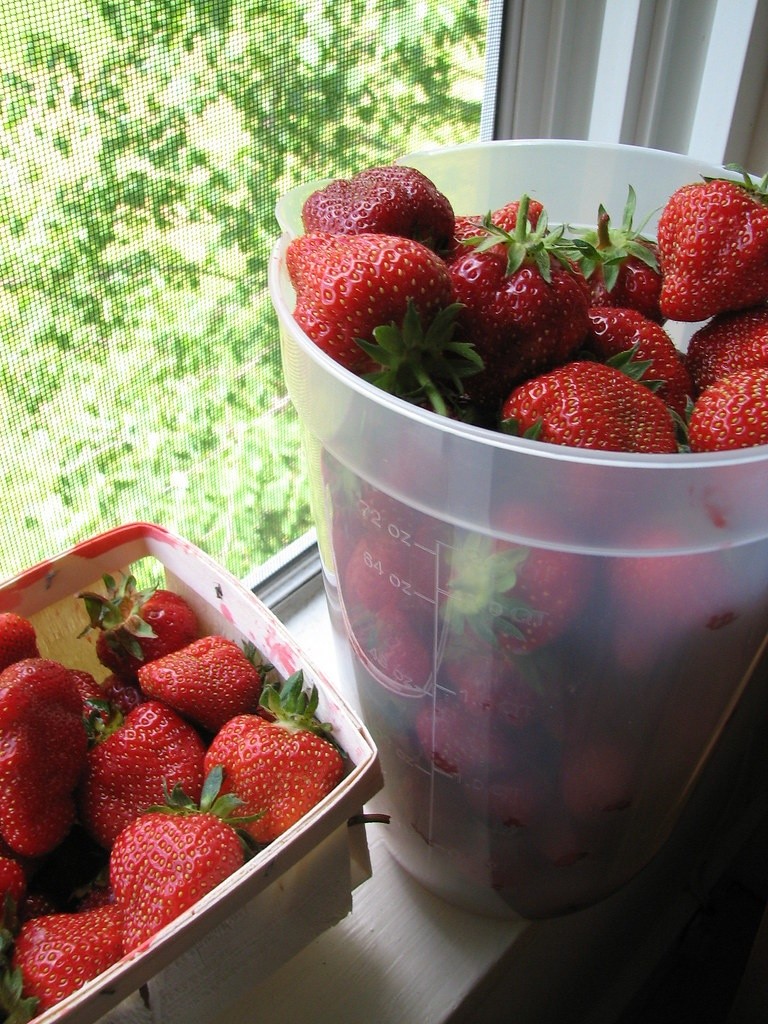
<box><xmin>264</xmin><ymin>137</ymin><xmax>766</xmax><ymax>921</ymax></box>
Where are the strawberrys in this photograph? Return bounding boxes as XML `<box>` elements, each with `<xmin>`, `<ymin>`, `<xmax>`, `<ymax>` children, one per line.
<box><xmin>0</xmin><ymin>569</ymin><xmax>345</xmax><ymax>1024</ymax></box>
<box><xmin>285</xmin><ymin>158</ymin><xmax>768</xmax><ymax>893</ymax></box>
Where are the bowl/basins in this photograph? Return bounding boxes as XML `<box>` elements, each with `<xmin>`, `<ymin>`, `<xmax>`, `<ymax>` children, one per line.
<box><xmin>2</xmin><ymin>520</ymin><xmax>385</xmax><ymax>1024</ymax></box>
